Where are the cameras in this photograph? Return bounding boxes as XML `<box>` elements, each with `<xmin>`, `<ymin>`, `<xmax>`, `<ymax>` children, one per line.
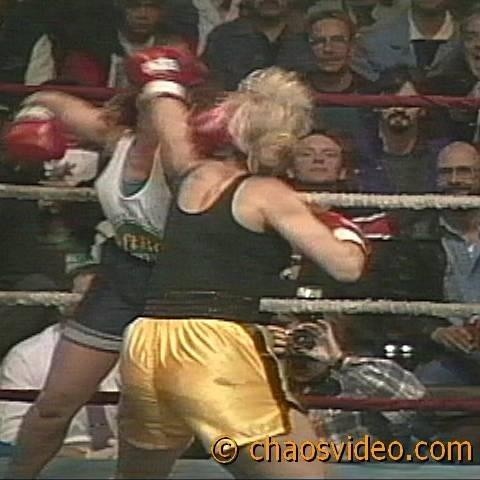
<box><xmin>282</xmin><ymin>330</ymin><xmax>314</xmax><ymax>356</ymax></box>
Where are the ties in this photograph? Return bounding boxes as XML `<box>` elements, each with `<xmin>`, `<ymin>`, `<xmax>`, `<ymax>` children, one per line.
<box><xmin>87</xmin><ymin>380</ymin><xmax>113</xmax><ymax>453</ymax></box>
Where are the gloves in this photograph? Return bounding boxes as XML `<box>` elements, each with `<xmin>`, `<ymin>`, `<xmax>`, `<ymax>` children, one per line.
<box><xmin>127</xmin><ymin>46</ymin><xmax>196</xmax><ymax>101</ymax></box>
<box><xmin>191</xmin><ymin>104</ymin><xmax>234</xmax><ymax>152</ymax></box>
<box><xmin>10</xmin><ymin>105</ymin><xmax>67</xmax><ymax>162</ymax></box>
<box><xmin>316</xmin><ymin>211</ymin><xmax>367</xmax><ymax>262</ymax></box>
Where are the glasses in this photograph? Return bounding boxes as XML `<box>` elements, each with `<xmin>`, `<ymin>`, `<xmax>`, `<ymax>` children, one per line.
<box><xmin>434</xmin><ymin>164</ymin><xmax>480</xmax><ymax>180</ymax></box>
